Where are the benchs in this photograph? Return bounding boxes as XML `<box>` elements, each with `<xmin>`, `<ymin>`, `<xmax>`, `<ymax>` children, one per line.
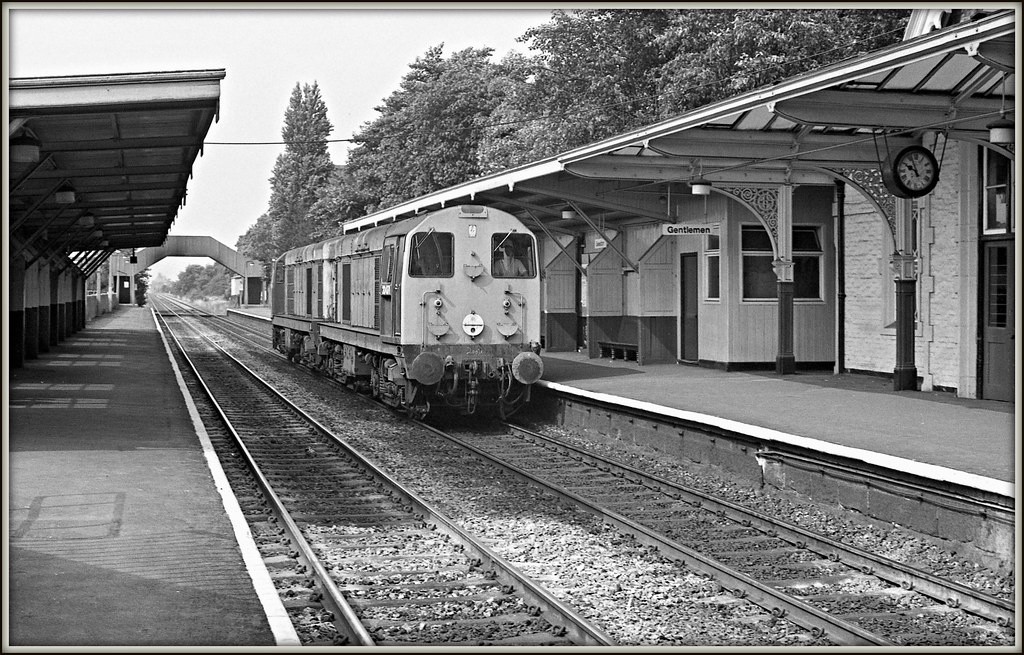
<box><xmin>597</xmin><ymin>341</ymin><xmax>640</xmax><ymax>363</ymax></box>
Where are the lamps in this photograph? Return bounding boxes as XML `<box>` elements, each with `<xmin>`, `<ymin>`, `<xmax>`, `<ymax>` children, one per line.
<box><xmin>986</xmin><ymin>72</ymin><xmax>1015</xmax><ymax>144</ymax></box>
<box><xmin>80</xmin><ymin>211</ymin><xmax>95</xmax><ymax>228</ymax></box>
<box><xmin>9</xmin><ymin>123</ymin><xmax>43</xmax><ymax>163</ymax></box>
<box><xmin>690</xmin><ymin>158</ymin><xmax>712</xmax><ymax>195</ymax></box>
<box><xmin>55</xmin><ymin>183</ymin><xmax>76</xmax><ymax>204</ymax></box>
<box><xmin>100</xmin><ymin>238</ymin><xmax>109</xmax><ymax>246</ymax></box>
<box><xmin>560</xmin><ymin>204</ymin><xmax>576</xmax><ymax>219</ymax></box>
<box><xmin>94</xmin><ymin>227</ymin><xmax>102</xmax><ymax>237</ymax></box>
<box><xmin>130</xmin><ymin>248</ymin><xmax>137</xmax><ymax>263</ymax></box>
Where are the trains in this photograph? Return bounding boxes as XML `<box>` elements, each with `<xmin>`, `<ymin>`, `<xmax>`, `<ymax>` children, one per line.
<box><xmin>268</xmin><ymin>203</ymin><xmax>542</xmax><ymax>424</ymax></box>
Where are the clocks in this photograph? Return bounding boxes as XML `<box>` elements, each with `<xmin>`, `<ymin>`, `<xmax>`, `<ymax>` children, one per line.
<box><xmin>872</xmin><ymin>128</ymin><xmax>949</xmax><ymax>199</ymax></box>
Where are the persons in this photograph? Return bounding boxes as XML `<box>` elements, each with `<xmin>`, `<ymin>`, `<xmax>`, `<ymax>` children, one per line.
<box><xmin>495</xmin><ymin>246</ymin><xmax>529</xmax><ymax>277</ymax></box>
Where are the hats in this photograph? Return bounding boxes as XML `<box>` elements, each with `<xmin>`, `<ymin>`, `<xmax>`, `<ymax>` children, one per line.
<box><xmin>502</xmin><ymin>239</ymin><xmax>513</xmax><ymax>247</ymax></box>
